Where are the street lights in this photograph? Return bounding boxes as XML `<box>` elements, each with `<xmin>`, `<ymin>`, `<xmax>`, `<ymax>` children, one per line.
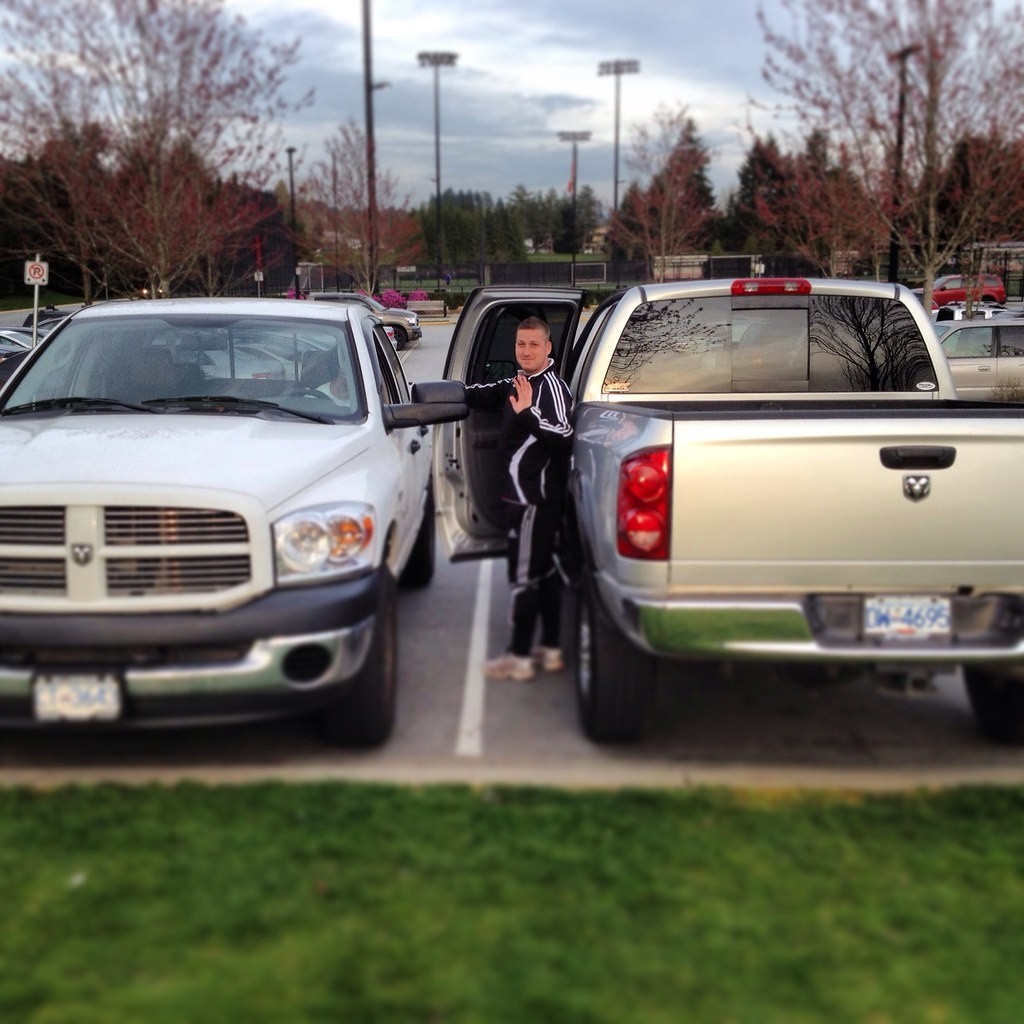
<box><xmin>885</xmin><ymin>44</ymin><xmax>926</xmax><ymax>283</ymax></box>
<box><xmin>284</xmin><ymin>145</ymin><xmax>301</xmax><ymax>301</ymax></box>
<box><xmin>417</xmin><ymin>48</ymin><xmax>459</xmax><ymax>291</ymax></box>
<box><xmin>598</xmin><ymin>55</ymin><xmax>640</xmax><ymax>212</ymax></box>
<box><xmin>557</xmin><ymin>130</ymin><xmax>594</xmax><ymax>255</ymax></box>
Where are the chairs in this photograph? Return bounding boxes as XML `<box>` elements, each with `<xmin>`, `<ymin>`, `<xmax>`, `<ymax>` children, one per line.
<box><xmin>289</xmin><ymin>348</ymin><xmax>338</xmax><ymax>398</ymax></box>
<box><xmin>104</xmin><ymin>344</ymin><xmax>186</xmax><ymax>401</ymax></box>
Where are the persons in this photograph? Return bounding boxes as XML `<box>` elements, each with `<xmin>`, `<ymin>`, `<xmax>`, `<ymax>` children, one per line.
<box><xmin>303</xmin><ymin>344</ymin><xmax>350</xmax><ymax>407</ymax></box>
<box><xmin>466</xmin><ymin>314</ymin><xmax>577</xmax><ymax>683</ymax></box>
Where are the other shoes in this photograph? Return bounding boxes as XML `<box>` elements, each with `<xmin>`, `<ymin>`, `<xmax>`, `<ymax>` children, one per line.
<box><xmin>481</xmin><ymin>653</ymin><xmax>535</xmax><ymax>680</ymax></box>
<box><xmin>529</xmin><ymin>642</ymin><xmax>563</xmax><ymax>671</ymax></box>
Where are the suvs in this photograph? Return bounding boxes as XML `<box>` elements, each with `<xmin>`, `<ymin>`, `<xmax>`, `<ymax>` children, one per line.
<box><xmin>905</xmin><ymin>273</ymin><xmax>1007</xmax><ymax>310</ymax></box>
<box><xmin>307</xmin><ymin>291</ymin><xmax>422</xmax><ymax>352</ymax></box>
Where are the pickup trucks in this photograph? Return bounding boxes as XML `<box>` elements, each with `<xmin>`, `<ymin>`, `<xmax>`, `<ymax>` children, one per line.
<box><xmin>0</xmin><ymin>296</ymin><xmax>467</xmax><ymax>751</ymax></box>
<box><xmin>428</xmin><ymin>278</ymin><xmax>1023</xmax><ymax>748</ymax></box>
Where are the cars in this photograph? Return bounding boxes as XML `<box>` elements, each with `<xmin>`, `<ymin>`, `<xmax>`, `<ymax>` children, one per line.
<box><xmin>21</xmin><ymin>303</ymin><xmax>76</xmax><ymax>331</ymax></box>
<box><xmin>932</xmin><ymin>318</ymin><xmax>1024</xmax><ymax>403</ymax></box>
<box><xmin>929</xmin><ymin>302</ymin><xmax>1024</xmax><ymax>320</ymax></box>
<box><xmin>0</xmin><ymin>325</ymin><xmax>51</xmax><ymax>388</ymax></box>
